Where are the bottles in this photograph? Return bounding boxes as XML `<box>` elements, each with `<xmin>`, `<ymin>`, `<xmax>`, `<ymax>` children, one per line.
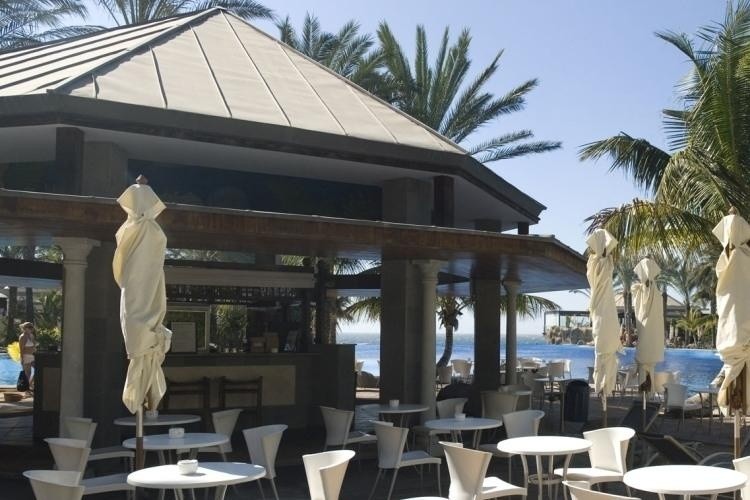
<box><xmin>223</xmin><ymin>342</ymin><xmax>245</xmax><ymax>353</ymax></box>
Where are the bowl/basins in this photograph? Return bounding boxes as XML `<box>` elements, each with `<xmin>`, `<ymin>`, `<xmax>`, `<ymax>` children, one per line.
<box><xmin>147</xmin><ymin>411</ymin><xmax>159</xmax><ymax>417</ymax></box>
<box><xmin>177</xmin><ymin>460</ymin><xmax>197</xmax><ymax>476</ymax></box>
<box><xmin>455</xmin><ymin>414</ymin><xmax>465</xmax><ymax>422</ymax></box>
<box><xmin>168</xmin><ymin>428</ymin><xmax>184</xmax><ymax>437</ymax></box>
<box><xmin>389</xmin><ymin>399</ymin><xmax>400</xmax><ymax>406</ymax></box>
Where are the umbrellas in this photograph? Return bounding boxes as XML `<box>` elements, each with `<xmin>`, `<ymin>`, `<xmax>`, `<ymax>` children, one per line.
<box><xmin>710</xmin><ymin>206</ymin><xmax>750</xmax><ymax>458</ymax></box>
<box><xmin>584</xmin><ymin>225</ymin><xmax>622</xmax><ymax>427</ymax></box>
<box><xmin>110</xmin><ymin>172</ymin><xmax>173</xmax><ymax>472</ymax></box>
<box><xmin>629</xmin><ymin>258</ymin><xmax>666</xmax><ymax>467</ymax></box>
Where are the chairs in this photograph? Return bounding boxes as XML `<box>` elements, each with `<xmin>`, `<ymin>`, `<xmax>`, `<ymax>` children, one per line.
<box><xmin>173</xmin><ymin>409</ymin><xmax>241</xmax><ymax>494</ymax></box>
<box><xmin>561</xmin><ymin>480</ymin><xmax>640</xmax><ymax>500</ymax></box>
<box><xmin>563</xmin><ymin>401</ymin><xmax>664</xmax><ymax>467</ymax></box>
<box><xmin>22</xmin><ymin>470</ymin><xmax>85</xmax><ymax>500</ymax></box>
<box><xmin>302</xmin><ymin>449</ymin><xmax>356</xmax><ymax>498</ymax></box>
<box><xmin>553</xmin><ymin>426</ymin><xmax>635</xmax><ymax>498</ymax></box>
<box><xmin>733</xmin><ymin>457</ymin><xmax>749</xmax><ymax>500</ymax></box>
<box><xmin>355</xmin><ymin>357</ymin><xmax>746</xmax><ymax>436</ymax></box>
<box><xmin>368</xmin><ymin>419</ymin><xmax>444</xmax><ymax>499</ymax></box>
<box><xmin>319</xmin><ymin>406</ymin><xmax>386</xmax><ymax>486</ymax></box>
<box><xmin>639</xmin><ymin>433</ymin><xmax>738</xmax><ymax>499</ymax></box>
<box><xmin>228</xmin><ymin>424</ymin><xmax>287</xmax><ymax>499</ymax></box>
<box><xmin>479</xmin><ymin>409</ymin><xmax>543</xmax><ymax>483</ymax></box>
<box><xmin>436</xmin><ymin>440</ymin><xmax>527</xmax><ymax>499</ymax></box>
<box><xmin>63</xmin><ymin>416</ymin><xmax>128</xmax><ymax>478</ymax></box>
<box><xmin>47</xmin><ymin>438</ymin><xmax>135</xmax><ymax>496</ymax></box>
<box><xmin>411</xmin><ymin>397</ymin><xmax>467</xmax><ymax>472</ymax></box>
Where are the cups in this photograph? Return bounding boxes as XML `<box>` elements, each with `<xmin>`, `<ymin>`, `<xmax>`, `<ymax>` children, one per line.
<box><xmin>549</xmin><ymin>374</ymin><xmax>554</xmax><ymax>380</ymax></box>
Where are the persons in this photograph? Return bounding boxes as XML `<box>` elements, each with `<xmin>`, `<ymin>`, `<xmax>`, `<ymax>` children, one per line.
<box><xmin>18</xmin><ymin>322</ymin><xmax>36</xmax><ymax>396</ymax></box>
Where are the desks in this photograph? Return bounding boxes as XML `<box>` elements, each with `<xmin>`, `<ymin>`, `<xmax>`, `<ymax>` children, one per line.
<box><xmin>363</xmin><ymin>403</ymin><xmax>427</xmax><ymax>477</ymax></box>
<box><xmin>127</xmin><ymin>461</ymin><xmax>265</xmax><ymax>498</ymax></box>
<box><xmin>114</xmin><ymin>414</ymin><xmax>201</xmax><ymax>468</ymax></box>
<box><xmin>423</xmin><ymin>418</ymin><xmax>502</xmax><ymax>450</ymax></box>
<box><xmin>624</xmin><ymin>463</ymin><xmax>749</xmax><ymax>498</ymax></box>
<box><xmin>500</xmin><ymin>435</ymin><xmax>592</xmax><ymax>499</ymax></box>
<box><xmin>123</xmin><ymin>431</ymin><xmax>232</xmax><ymax>500</ymax></box>
